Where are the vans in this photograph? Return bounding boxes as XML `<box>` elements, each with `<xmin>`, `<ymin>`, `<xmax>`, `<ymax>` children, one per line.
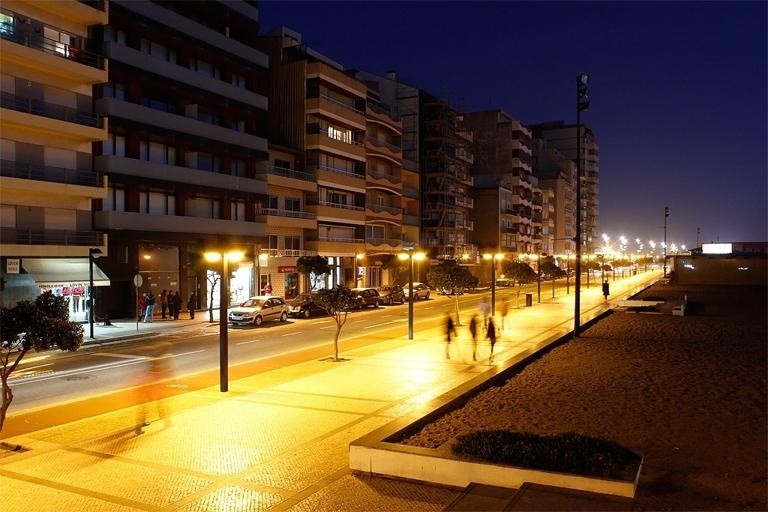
<box><xmin>337</xmin><ymin>287</ymin><xmax>381</xmax><ymax>310</ymax></box>
<box><xmin>376</xmin><ymin>284</ymin><xmax>406</xmax><ymax>305</ymax></box>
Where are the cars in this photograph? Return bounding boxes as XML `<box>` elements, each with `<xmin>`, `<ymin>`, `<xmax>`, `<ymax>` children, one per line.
<box><xmin>229</xmin><ymin>294</ymin><xmax>290</xmax><ymax>326</ymax></box>
<box><xmin>287</xmin><ymin>291</ymin><xmax>336</xmax><ymax>319</ymax></box>
<box><xmin>402</xmin><ymin>281</ymin><xmax>431</xmax><ymax>301</ymax></box>
<box><xmin>497</xmin><ymin>273</ymin><xmax>516</xmax><ymax>287</ymax></box>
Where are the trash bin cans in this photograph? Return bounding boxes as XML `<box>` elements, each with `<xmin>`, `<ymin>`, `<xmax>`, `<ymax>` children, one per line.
<box><xmin>526</xmin><ymin>294</ymin><xmax>532</xmax><ymax>306</ymax></box>
<box><xmin>633</xmin><ymin>271</ymin><xmax>636</xmax><ymax>275</ymax></box>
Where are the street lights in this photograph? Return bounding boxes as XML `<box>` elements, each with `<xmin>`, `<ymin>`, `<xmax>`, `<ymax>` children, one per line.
<box><xmin>528</xmin><ymin>251</ymin><xmax>549</xmax><ymax>303</ymax></box>
<box><xmin>480</xmin><ymin>250</ymin><xmax>505</xmax><ymax>317</ymax></box>
<box><xmin>353</xmin><ymin>248</ymin><xmax>366</xmax><ymax>288</ymax></box>
<box><xmin>561</xmin><ymin>251</ymin><xmax>651</xmax><ymax>295</ymax></box>
<box><xmin>88</xmin><ymin>246</ymin><xmax>103</xmax><ymax>338</ymax></box>
<box><xmin>396</xmin><ymin>248</ymin><xmax>427</xmax><ymax>341</ymax></box>
<box><xmin>201</xmin><ymin>238</ymin><xmax>246</xmax><ymax>393</ymax></box>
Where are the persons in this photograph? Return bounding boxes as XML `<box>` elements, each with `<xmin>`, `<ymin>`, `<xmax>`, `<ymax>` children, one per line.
<box><xmin>469</xmin><ymin>295</ymin><xmax>508</xmax><ymax>362</ymax></box>
<box><xmin>602</xmin><ymin>279</ymin><xmax>610</xmax><ymax>301</ymax></box>
<box><xmin>263</xmin><ymin>281</ymin><xmax>272</xmax><ymax>296</ymax></box>
<box><xmin>71</xmin><ymin>292</ymin><xmax>85</xmax><ymax>313</ymax></box>
<box><xmin>441</xmin><ymin>312</ymin><xmax>458</xmax><ymax>359</ymax></box>
<box><xmin>138</xmin><ymin>290</ymin><xmax>196</xmax><ymax>323</ymax></box>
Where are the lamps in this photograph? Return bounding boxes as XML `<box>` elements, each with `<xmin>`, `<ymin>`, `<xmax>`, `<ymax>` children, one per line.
<box><xmin>144</xmin><ymin>248</ymin><xmax>152</xmax><ymax>260</ymax></box>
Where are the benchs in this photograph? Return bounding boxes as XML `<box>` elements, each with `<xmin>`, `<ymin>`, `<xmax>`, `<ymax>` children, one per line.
<box><xmin>671</xmin><ymin>293</ymin><xmax>688</xmax><ymax>316</ymax></box>
